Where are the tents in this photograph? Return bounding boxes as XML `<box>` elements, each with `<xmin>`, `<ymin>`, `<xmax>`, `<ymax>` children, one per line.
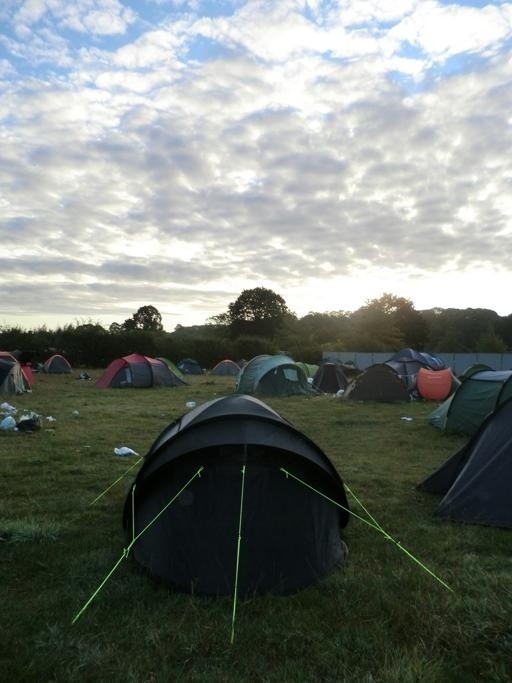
<box><xmin>414</xmin><ymin>374</ymin><xmax>512</xmax><ymax>529</ymax></box>
<box><xmin>176</xmin><ymin>358</ymin><xmax>201</xmax><ymax>374</ymax></box>
<box><xmin>122</xmin><ymin>393</ymin><xmax>350</xmax><ymax>600</ymax></box>
<box><xmin>344</xmin><ymin>348</ymin><xmax>495</xmax><ymax>403</ymax></box>
<box><xmin>93</xmin><ymin>352</ymin><xmax>189</xmax><ymax>386</ymax></box>
<box><xmin>428</xmin><ymin>370</ymin><xmax>512</xmax><ymax>434</ymax></box>
<box><xmin>1</xmin><ymin>351</ymin><xmax>31</xmax><ymax>393</ymax></box>
<box><xmin>236</xmin><ymin>354</ymin><xmax>359</xmax><ymax>398</ymax></box>
<box><xmin>211</xmin><ymin>360</ymin><xmax>241</xmax><ymax>376</ymax></box>
<box><xmin>40</xmin><ymin>355</ymin><xmax>71</xmax><ymax>373</ymax></box>
